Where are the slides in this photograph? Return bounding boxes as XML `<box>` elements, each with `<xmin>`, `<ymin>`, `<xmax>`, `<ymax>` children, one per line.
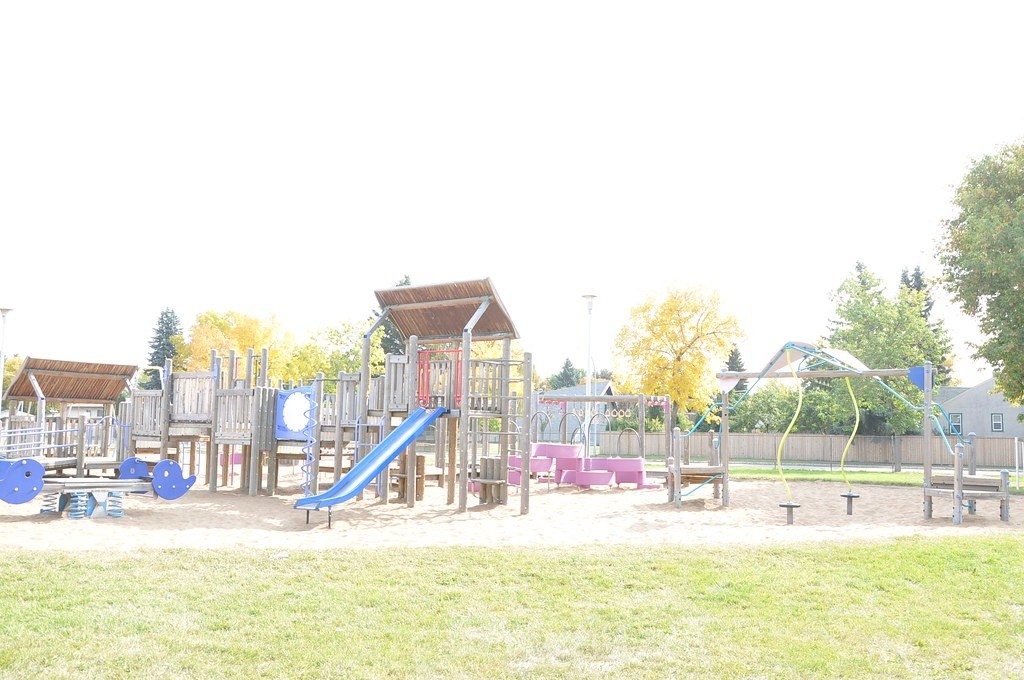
<box><xmin>293</xmin><ymin>406</ymin><xmax>448</xmax><ymax>511</ymax></box>
